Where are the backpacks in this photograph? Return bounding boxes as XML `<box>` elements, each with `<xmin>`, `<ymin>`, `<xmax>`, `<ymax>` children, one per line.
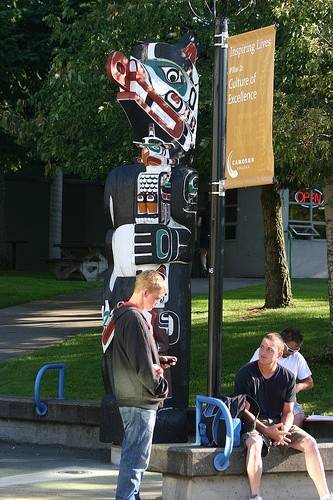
<box><xmin>198</xmin><ymin>394</ymin><xmax>260</xmax><ymax>448</ymax></box>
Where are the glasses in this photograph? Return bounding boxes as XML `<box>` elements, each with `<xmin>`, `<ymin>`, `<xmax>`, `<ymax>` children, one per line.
<box><xmin>285</xmin><ymin>342</ymin><xmax>300</xmax><ymax>353</ymax></box>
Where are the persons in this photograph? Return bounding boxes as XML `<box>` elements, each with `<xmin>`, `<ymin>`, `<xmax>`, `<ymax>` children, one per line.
<box><xmin>101</xmin><ymin>269</ymin><xmax>177</xmax><ymax>500</ymax></box>
<box><xmin>197</xmin><ymin>200</ymin><xmax>211</xmax><ymax>278</ymax></box>
<box><xmin>233</xmin><ymin>331</ymin><xmax>333</xmax><ymax>500</ymax></box>
<box><xmin>250</xmin><ymin>326</ymin><xmax>313</xmax><ymax>428</ymax></box>
